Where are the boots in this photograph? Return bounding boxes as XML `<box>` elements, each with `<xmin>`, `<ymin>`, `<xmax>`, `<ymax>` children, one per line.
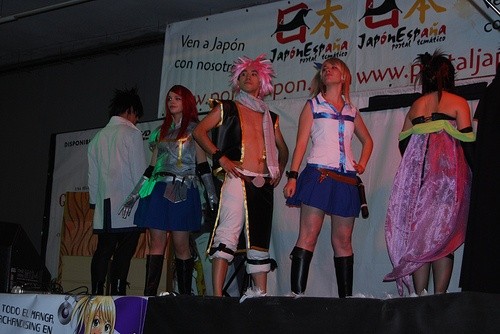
<box><xmin>111</xmin><ymin>278</ymin><xmax>130</xmax><ymax>297</ymax></box>
<box><xmin>91</xmin><ymin>281</ymin><xmax>104</xmax><ymax>296</ymax></box>
<box><xmin>288</xmin><ymin>246</ymin><xmax>313</xmax><ymax>298</ymax></box>
<box><xmin>333</xmin><ymin>253</ymin><xmax>355</xmax><ymax>299</ymax></box>
<box><xmin>175</xmin><ymin>257</ymin><xmax>193</xmax><ymax>296</ymax></box>
<box><xmin>143</xmin><ymin>254</ymin><xmax>167</xmax><ymax>296</ymax></box>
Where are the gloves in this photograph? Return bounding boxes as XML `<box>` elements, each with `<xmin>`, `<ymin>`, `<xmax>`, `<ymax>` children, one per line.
<box><xmin>201</xmin><ymin>173</ymin><xmax>219</xmax><ymax>210</ymax></box>
<box><xmin>117</xmin><ymin>175</ymin><xmax>148</xmax><ymax>219</ymax></box>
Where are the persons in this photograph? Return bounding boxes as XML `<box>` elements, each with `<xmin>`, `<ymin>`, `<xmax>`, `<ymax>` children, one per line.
<box><xmin>193</xmin><ymin>54</ymin><xmax>289</xmax><ymax>297</ymax></box>
<box><xmin>117</xmin><ymin>85</ymin><xmax>219</xmax><ymax>297</ymax></box>
<box><xmin>385</xmin><ymin>49</ymin><xmax>476</xmax><ymax>297</ymax></box>
<box><xmin>88</xmin><ymin>90</ymin><xmax>149</xmax><ymax>296</ymax></box>
<box><xmin>282</xmin><ymin>57</ymin><xmax>373</xmax><ymax>297</ymax></box>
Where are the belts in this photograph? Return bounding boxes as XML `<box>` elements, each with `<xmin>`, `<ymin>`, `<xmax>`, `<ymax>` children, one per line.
<box><xmin>155</xmin><ymin>176</ymin><xmax>191</xmax><ymax>187</ymax></box>
<box><xmin>242</xmin><ymin>175</ymin><xmax>271</xmax><ymax>184</ymax></box>
<box><xmin>317</xmin><ymin>168</ymin><xmax>359</xmax><ymax>186</ymax></box>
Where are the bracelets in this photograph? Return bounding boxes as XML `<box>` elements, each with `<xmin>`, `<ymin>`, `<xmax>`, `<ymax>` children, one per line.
<box><xmin>213</xmin><ymin>150</ymin><xmax>224</xmax><ymax>160</ymax></box>
<box><xmin>287</xmin><ymin>170</ymin><xmax>298</xmax><ymax>180</ymax></box>
